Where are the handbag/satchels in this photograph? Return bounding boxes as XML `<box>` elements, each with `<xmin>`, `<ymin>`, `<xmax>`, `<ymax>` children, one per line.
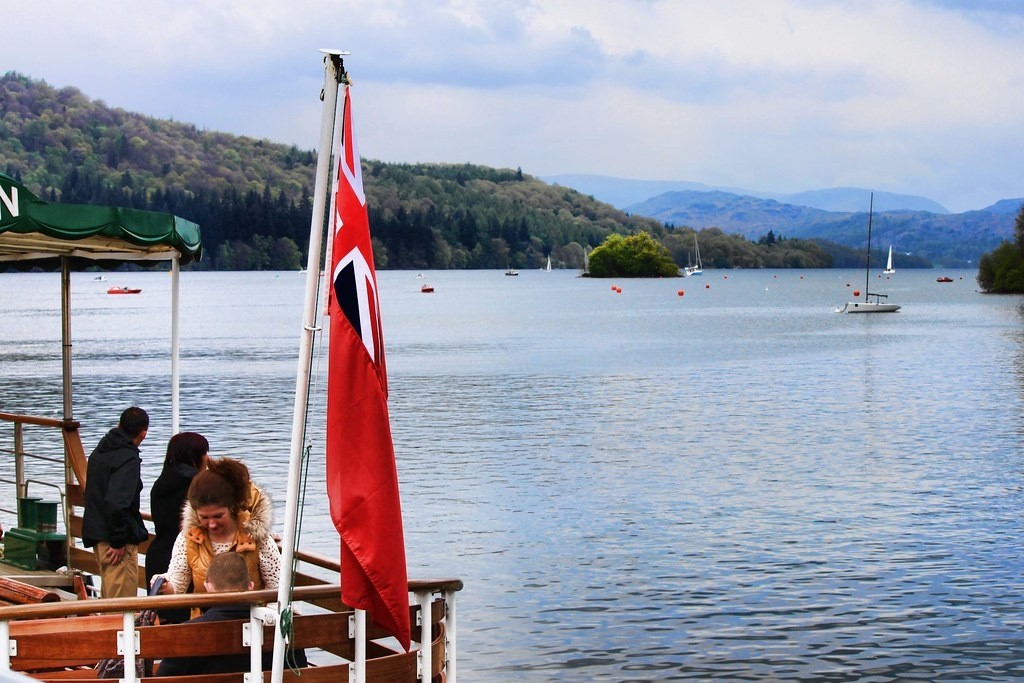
<box><xmin>93</xmin><ymin>576</ymin><xmax>167</xmax><ymax>679</ymax></box>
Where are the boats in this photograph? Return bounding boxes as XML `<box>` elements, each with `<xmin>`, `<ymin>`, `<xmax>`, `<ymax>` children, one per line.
<box><xmin>937</xmin><ymin>277</ymin><xmax>953</xmax><ymax>282</ymax></box>
<box><xmin>506</xmin><ymin>265</ymin><xmax>518</xmax><ymax>276</ymax></box>
<box><xmin>0</xmin><ymin>170</ymin><xmax>464</xmax><ymax>683</ymax></box>
<box><xmin>107</xmin><ymin>285</ymin><xmax>142</xmax><ymax>294</ymax></box>
<box><xmin>422</xmin><ymin>284</ymin><xmax>434</xmax><ymax>292</ymax></box>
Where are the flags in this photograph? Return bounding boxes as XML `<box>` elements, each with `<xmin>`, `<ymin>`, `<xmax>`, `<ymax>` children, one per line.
<box><xmin>327</xmin><ymin>88</ymin><xmax>412</xmax><ymax>662</ymax></box>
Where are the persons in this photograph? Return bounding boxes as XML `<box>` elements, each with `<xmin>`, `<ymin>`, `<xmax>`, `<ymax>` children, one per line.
<box><xmin>150</xmin><ymin>455</ymin><xmax>281</xmax><ymax>619</ymax></box>
<box><xmin>82</xmin><ymin>407</ymin><xmax>149</xmax><ymax>616</ymax></box>
<box><xmin>155</xmin><ymin>552</ymin><xmax>308</xmax><ymax>676</ymax></box>
<box><xmin>145</xmin><ymin>432</ymin><xmax>209</xmax><ymax>596</ymax></box>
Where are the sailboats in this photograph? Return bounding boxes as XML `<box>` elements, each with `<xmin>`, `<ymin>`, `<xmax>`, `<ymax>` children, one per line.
<box><xmin>685</xmin><ymin>234</ymin><xmax>703</xmax><ymax>276</ymax></box>
<box><xmin>839</xmin><ymin>191</ymin><xmax>901</xmax><ymax>313</ymax></box>
<box><xmin>584</xmin><ymin>248</ymin><xmax>590</xmax><ymax>274</ymax></box>
<box><xmin>884</xmin><ymin>244</ymin><xmax>896</xmax><ymax>274</ymax></box>
<box><xmin>544</xmin><ymin>255</ymin><xmax>551</xmax><ymax>272</ymax></box>
